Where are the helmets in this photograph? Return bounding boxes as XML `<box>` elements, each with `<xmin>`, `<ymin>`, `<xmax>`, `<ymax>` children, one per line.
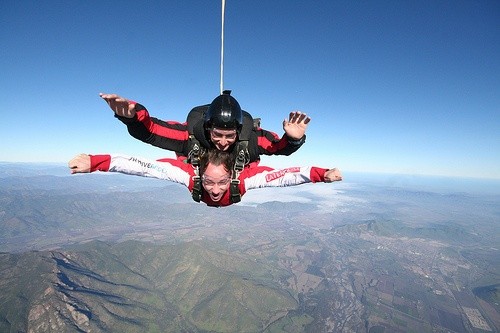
<box><xmin>208</xmin><ymin>94</ymin><xmax>242</xmax><ymax>132</ymax></box>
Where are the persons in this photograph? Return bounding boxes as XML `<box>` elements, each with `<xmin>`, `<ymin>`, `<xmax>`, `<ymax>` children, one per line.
<box><xmin>68</xmin><ymin>147</ymin><xmax>344</xmax><ymax>209</ymax></box>
<box><xmin>99</xmin><ymin>90</ymin><xmax>311</xmax><ymax>163</ymax></box>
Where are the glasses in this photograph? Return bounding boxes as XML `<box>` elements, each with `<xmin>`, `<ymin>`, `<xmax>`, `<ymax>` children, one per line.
<box><xmin>203</xmin><ymin>175</ymin><xmax>230</xmax><ymax>189</ymax></box>
<box><xmin>211</xmin><ymin>127</ymin><xmax>236</xmax><ymax>139</ymax></box>
